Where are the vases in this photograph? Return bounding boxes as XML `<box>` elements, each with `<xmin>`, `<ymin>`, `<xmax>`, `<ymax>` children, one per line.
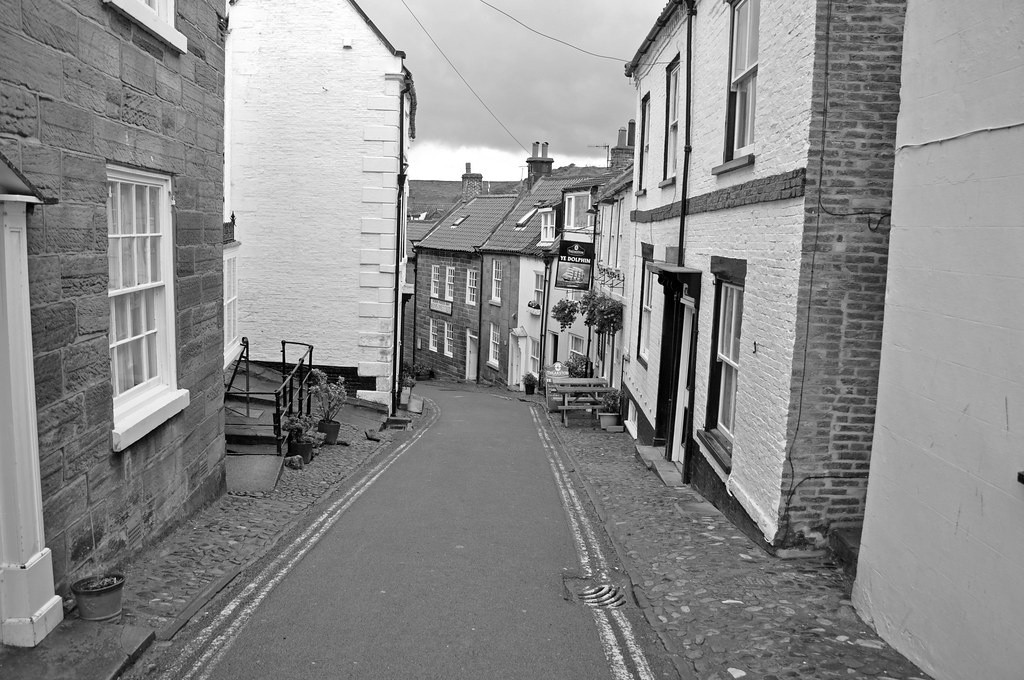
<box><xmin>70</xmin><ymin>573</ymin><xmax>127</xmax><ymax>625</ymax></box>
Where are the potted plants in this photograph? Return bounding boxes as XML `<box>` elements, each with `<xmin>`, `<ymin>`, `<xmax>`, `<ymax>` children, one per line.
<box><xmin>282</xmin><ymin>416</ymin><xmax>315</xmax><ymax>464</ymax></box>
<box><xmin>400</xmin><ymin>372</ymin><xmax>415</xmax><ymax>405</ymax></box>
<box><xmin>597</xmin><ymin>391</ymin><xmax>620</xmax><ymax>429</ymax></box>
<box><xmin>522</xmin><ymin>372</ymin><xmax>538</xmax><ymax>395</ymax></box>
<box><xmin>306</xmin><ymin>368</ymin><xmax>348</xmax><ymax>444</ymax></box>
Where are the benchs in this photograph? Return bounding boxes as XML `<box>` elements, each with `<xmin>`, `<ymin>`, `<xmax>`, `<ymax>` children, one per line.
<box><xmin>553</xmin><ymin>377</ymin><xmax>617</xmax><ymax>428</ymax></box>
